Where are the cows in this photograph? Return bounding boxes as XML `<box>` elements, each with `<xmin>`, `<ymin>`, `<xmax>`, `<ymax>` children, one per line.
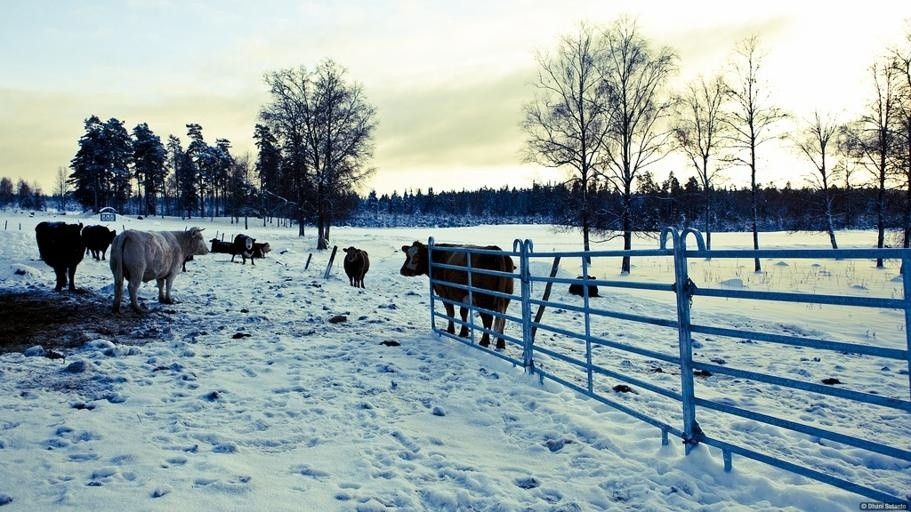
<box><xmin>253</xmin><ymin>242</ymin><xmax>271</xmax><ymax>259</ymax></box>
<box><xmin>35</xmin><ymin>221</ymin><xmax>86</xmax><ymax>293</ymax></box>
<box><xmin>343</xmin><ymin>246</ymin><xmax>370</xmax><ymax>290</ymax></box>
<box><xmin>209</xmin><ymin>238</ymin><xmax>235</xmax><ymax>255</ymax></box>
<box><xmin>81</xmin><ymin>224</ymin><xmax>118</xmax><ymax>261</ymax></box>
<box><xmin>110</xmin><ymin>226</ymin><xmax>211</xmax><ymax>311</ymax></box>
<box><xmin>399</xmin><ymin>241</ymin><xmax>515</xmax><ymax>350</ymax></box>
<box><xmin>231</xmin><ymin>234</ymin><xmax>256</xmax><ymax>265</ymax></box>
<box><xmin>568</xmin><ymin>274</ymin><xmax>601</xmax><ymax>299</ymax></box>
